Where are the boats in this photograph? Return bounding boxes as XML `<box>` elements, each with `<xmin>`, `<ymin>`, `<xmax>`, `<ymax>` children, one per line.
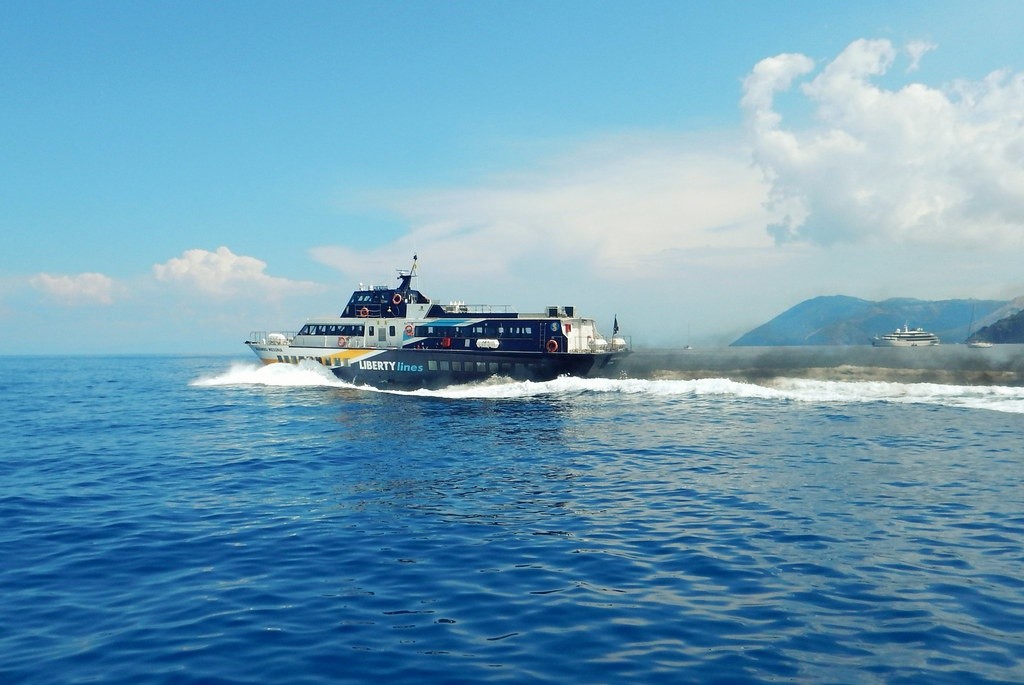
<box><xmin>243</xmin><ymin>254</ymin><xmax>633</xmax><ymax>391</ymax></box>
<box><xmin>969</xmin><ymin>340</ymin><xmax>993</xmax><ymax>349</ymax></box>
<box><xmin>871</xmin><ymin>324</ymin><xmax>940</xmax><ymax>349</ymax></box>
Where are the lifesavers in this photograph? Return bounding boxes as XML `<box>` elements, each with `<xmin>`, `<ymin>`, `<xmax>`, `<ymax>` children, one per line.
<box><xmin>546</xmin><ymin>339</ymin><xmax>558</xmax><ymax>352</ymax></box>
<box><xmin>360</xmin><ymin>307</ymin><xmax>369</xmax><ymax>318</ymax></box>
<box><xmin>405</xmin><ymin>324</ymin><xmax>414</xmax><ymax>336</ymax></box>
<box><xmin>337</xmin><ymin>336</ymin><xmax>347</xmax><ymax>347</ymax></box>
<box><xmin>392</xmin><ymin>294</ymin><xmax>402</xmax><ymax>305</ymax></box>
